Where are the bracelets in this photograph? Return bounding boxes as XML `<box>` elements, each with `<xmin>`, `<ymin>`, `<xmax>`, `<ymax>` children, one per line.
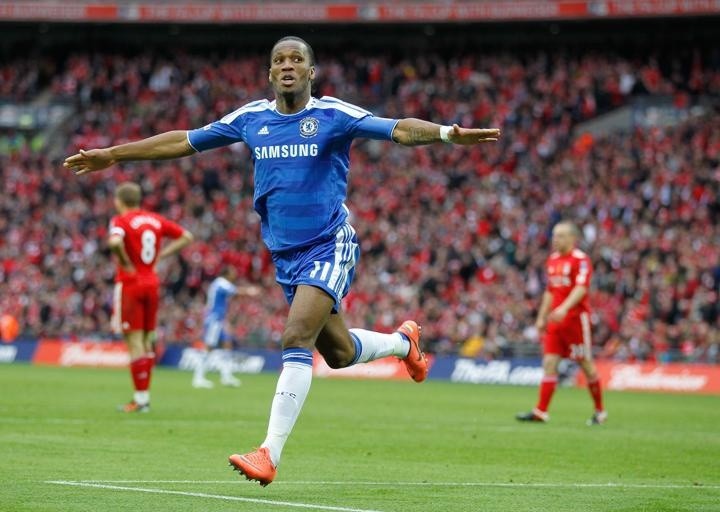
<box><xmin>439</xmin><ymin>124</ymin><xmax>453</xmax><ymax>145</ymax></box>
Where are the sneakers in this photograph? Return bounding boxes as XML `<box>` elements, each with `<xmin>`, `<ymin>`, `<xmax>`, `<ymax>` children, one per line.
<box><xmin>589</xmin><ymin>412</ymin><xmax>606</xmax><ymax>425</ymax></box>
<box><xmin>517</xmin><ymin>412</ymin><xmax>545</xmax><ymax>423</ymax></box>
<box><xmin>115</xmin><ymin>401</ymin><xmax>148</xmax><ymax>414</ymax></box>
<box><xmin>397</xmin><ymin>320</ymin><xmax>427</xmax><ymax>382</ymax></box>
<box><xmin>229</xmin><ymin>450</ymin><xmax>278</xmax><ymax>486</ymax></box>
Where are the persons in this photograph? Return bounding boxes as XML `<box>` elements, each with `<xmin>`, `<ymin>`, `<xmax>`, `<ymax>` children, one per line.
<box><xmin>309</xmin><ymin>48</ymin><xmax>720</xmax><ymax>368</ymax></box>
<box><xmin>515</xmin><ymin>216</ymin><xmax>610</xmax><ymax>426</ymax></box>
<box><xmin>1</xmin><ymin>48</ymin><xmax>289</xmax><ymax>344</ymax></box>
<box><xmin>66</xmin><ymin>36</ymin><xmax>502</xmax><ymax>488</ymax></box>
<box><xmin>191</xmin><ymin>264</ymin><xmax>263</xmax><ymax>388</ymax></box>
<box><xmin>105</xmin><ymin>181</ymin><xmax>191</xmax><ymax>415</ymax></box>
